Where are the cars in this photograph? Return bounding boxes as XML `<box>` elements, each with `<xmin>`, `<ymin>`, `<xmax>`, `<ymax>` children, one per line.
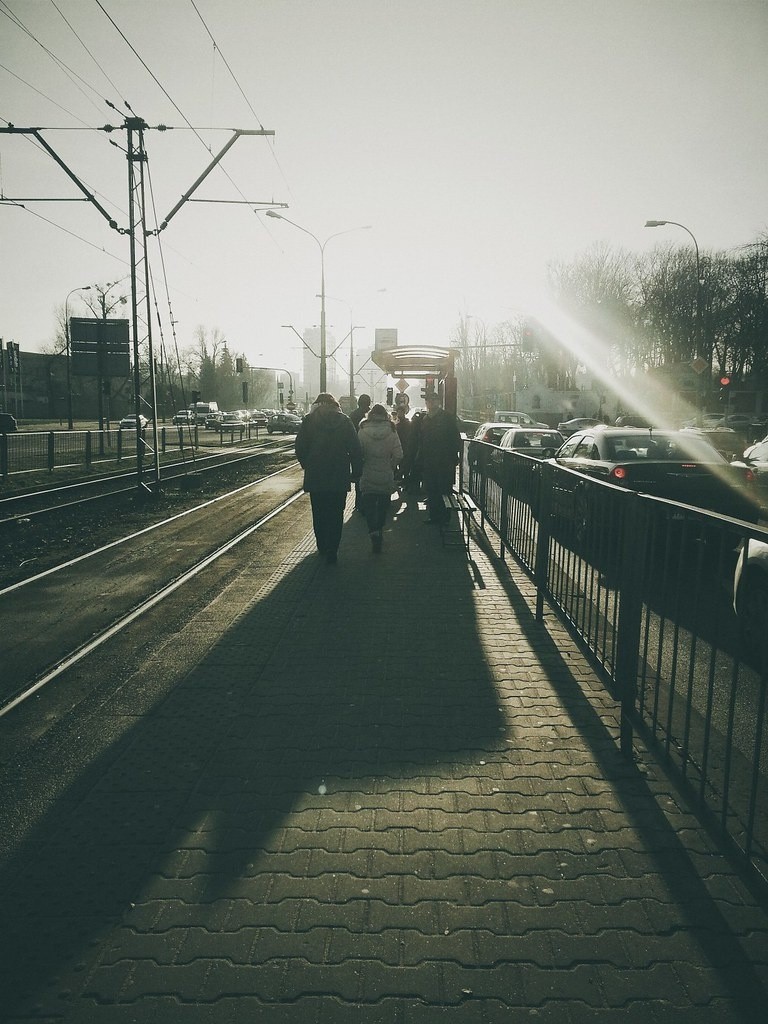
<box><xmin>473</xmin><ymin>423</ymin><xmax>531</xmax><ymax>449</ymax></box>
<box><xmin>538</xmin><ymin>429</ymin><xmax>761</xmax><ymax>551</ymax></box>
<box><xmin>495</xmin><ymin>429</ymin><xmax>566</xmax><ymax>456</ymax></box>
<box><xmin>119</xmin><ymin>414</ymin><xmax>148</xmax><ymax>429</ymax></box>
<box><xmin>456</xmin><ymin>415</ymin><xmax>480</xmax><ymax>436</ymax></box>
<box><xmin>615</xmin><ymin>412</ymin><xmax>768</xmax><ymax>494</ymax></box>
<box><xmin>557</xmin><ymin>418</ymin><xmax>606</xmax><ymax>438</ymax></box>
<box><xmin>173</xmin><ymin>402</ymin><xmax>303</xmax><ymax>435</ymax></box>
<box><xmin>494</xmin><ymin>411</ymin><xmax>549</xmax><ymax>429</ymax></box>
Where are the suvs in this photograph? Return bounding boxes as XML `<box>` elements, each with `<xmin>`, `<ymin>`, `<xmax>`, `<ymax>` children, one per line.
<box><xmin>0</xmin><ymin>413</ymin><xmax>18</xmax><ymax>432</ymax></box>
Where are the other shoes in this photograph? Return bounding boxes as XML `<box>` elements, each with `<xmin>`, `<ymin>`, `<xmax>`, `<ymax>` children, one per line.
<box><xmin>318</xmin><ymin>542</ymin><xmax>327</xmax><ymax>554</ymax></box>
<box><xmin>327</xmin><ymin>547</ymin><xmax>338</xmax><ymax>564</ymax></box>
<box><xmin>423</xmin><ymin>517</ymin><xmax>435</xmax><ymax>523</ymax></box>
<box><xmin>369</xmin><ymin>528</ymin><xmax>382</xmax><ymax>554</ymax></box>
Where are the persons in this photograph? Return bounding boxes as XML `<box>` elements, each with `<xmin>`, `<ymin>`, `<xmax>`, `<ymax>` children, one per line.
<box><xmin>347</xmin><ymin>392</ymin><xmax>462</xmax><ymax>550</ymax></box>
<box><xmin>295</xmin><ymin>393</ymin><xmax>363</xmax><ymax>563</ymax></box>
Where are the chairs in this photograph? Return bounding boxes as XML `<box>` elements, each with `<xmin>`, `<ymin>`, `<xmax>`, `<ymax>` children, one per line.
<box><xmin>517</xmin><ymin>438</ymin><xmax>531</xmax><ymax>447</ymax></box>
<box><xmin>540</xmin><ymin>436</ymin><xmax>553</xmax><ymax>447</ymax></box>
<box><xmin>617</xmin><ymin>450</ymin><xmax>637</xmax><ymax>459</ymax></box>
<box><xmin>647</xmin><ymin>446</ymin><xmax>668</xmax><ymax>458</ymax></box>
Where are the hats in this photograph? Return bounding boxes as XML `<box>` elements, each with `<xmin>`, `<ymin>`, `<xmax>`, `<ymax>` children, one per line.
<box><xmin>312</xmin><ymin>393</ymin><xmax>339</xmax><ymax>407</ymax></box>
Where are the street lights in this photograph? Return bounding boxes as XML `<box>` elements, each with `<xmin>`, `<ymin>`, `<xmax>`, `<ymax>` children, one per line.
<box><xmin>65</xmin><ymin>285</ymin><xmax>91</xmax><ymax>434</ymax></box>
<box><xmin>644</xmin><ymin>220</ymin><xmax>700</xmax><ymax>357</ymax></box>
<box><xmin>266</xmin><ymin>208</ymin><xmax>373</xmax><ymax>391</ymax></box>
<box><xmin>315</xmin><ymin>288</ymin><xmax>388</xmax><ymax>412</ymax></box>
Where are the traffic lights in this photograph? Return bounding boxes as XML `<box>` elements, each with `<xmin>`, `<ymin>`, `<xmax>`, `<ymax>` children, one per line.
<box><xmin>235</xmin><ymin>358</ymin><xmax>244</xmax><ymax>372</ymax></box>
